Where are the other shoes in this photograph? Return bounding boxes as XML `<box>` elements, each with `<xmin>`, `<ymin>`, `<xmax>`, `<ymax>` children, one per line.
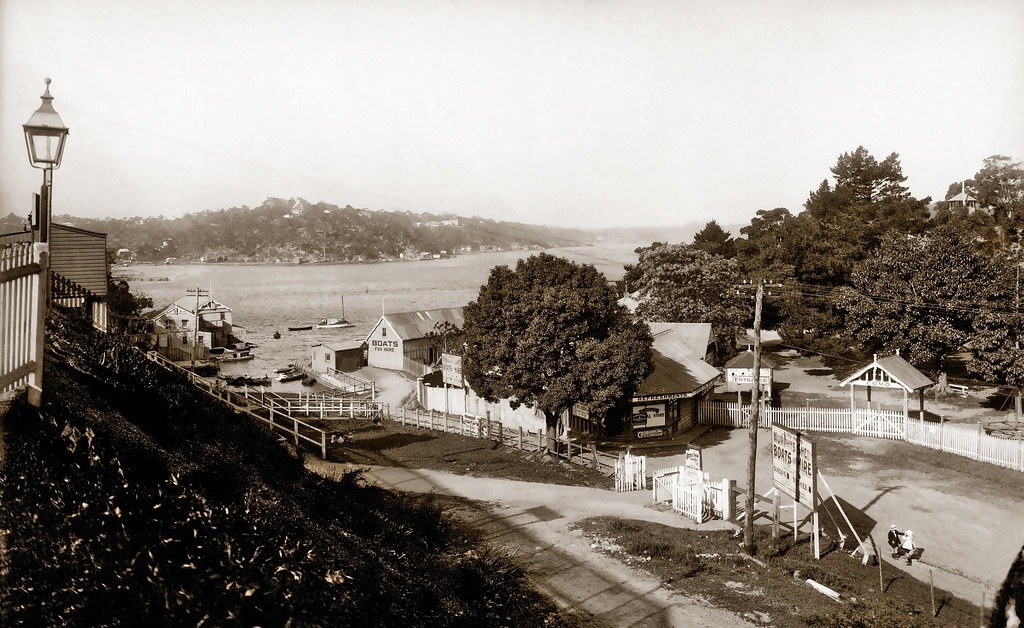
<box><xmin>906</xmin><ymin>560</ymin><xmax>912</xmax><ymax>566</ymax></box>
<box><xmin>892</xmin><ymin>553</ymin><xmax>900</xmax><ymax>558</ymax></box>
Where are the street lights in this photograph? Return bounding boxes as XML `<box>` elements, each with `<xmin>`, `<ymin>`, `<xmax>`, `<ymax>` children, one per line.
<box><xmin>23</xmin><ymin>77</ymin><xmax>70</xmax><ymax>243</ymax></box>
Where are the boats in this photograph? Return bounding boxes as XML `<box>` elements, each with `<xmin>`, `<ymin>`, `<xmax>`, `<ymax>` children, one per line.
<box><xmin>316</xmin><ymin>294</ymin><xmax>356</xmax><ymax>328</ymax></box>
<box><xmin>217</xmin><ymin>359</ymin><xmax>316</xmax><ymax>386</ymax></box>
<box><xmin>288</xmin><ymin>326</ymin><xmax>312</xmax><ymax>330</ymax></box>
<box><xmin>222</xmin><ymin>351</ymin><xmax>254</xmax><ymax>360</ymax></box>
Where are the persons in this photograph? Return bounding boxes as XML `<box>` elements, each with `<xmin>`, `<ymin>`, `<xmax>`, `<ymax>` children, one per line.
<box><xmin>888</xmin><ymin>524</ymin><xmax>905</xmax><ymax>560</ymax></box>
<box><xmin>900</xmin><ymin>530</ymin><xmax>914</xmax><ymax>566</ymax></box>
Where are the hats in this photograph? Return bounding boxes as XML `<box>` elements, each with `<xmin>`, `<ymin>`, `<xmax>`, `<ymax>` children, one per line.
<box><xmin>905</xmin><ymin>530</ymin><xmax>914</xmax><ymax>538</ymax></box>
<box><xmin>890</xmin><ymin>524</ymin><xmax>897</xmax><ymax>530</ymax></box>
